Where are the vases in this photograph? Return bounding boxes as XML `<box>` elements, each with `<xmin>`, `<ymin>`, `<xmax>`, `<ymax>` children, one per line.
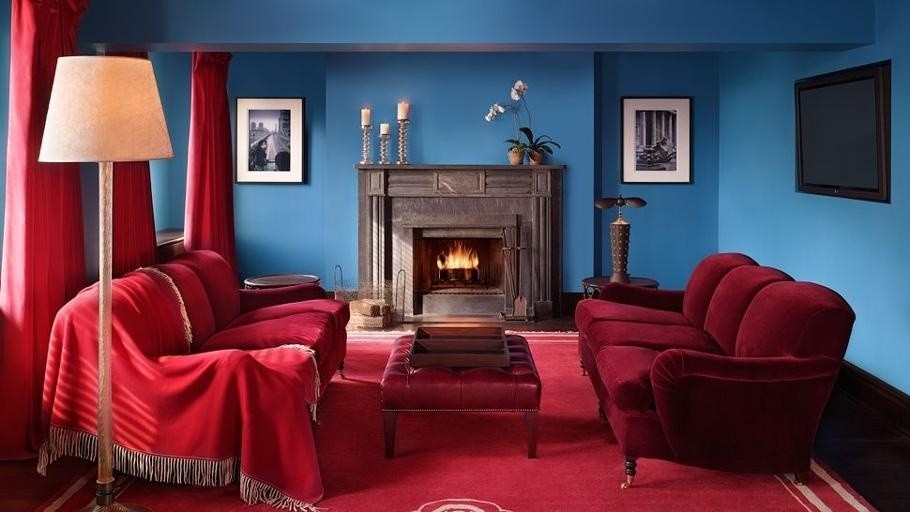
<box><xmin>529</xmin><ymin>151</ymin><xmax>543</xmax><ymax>166</ymax></box>
<box><xmin>508</xmin><ymin>148</ymin><xmax>525</xmax><ymax>165</ymax></box>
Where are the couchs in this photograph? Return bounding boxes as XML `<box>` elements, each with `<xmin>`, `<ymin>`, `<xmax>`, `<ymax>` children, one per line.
<box><xmin>573</xmin><ymin>252</ymin><xmax>857</xmax><ymax>486</ymax></box>
<box><xmin>37</xmin><ymin>249</ymin><xmax>350</xmax><ymax>512</ymax></box>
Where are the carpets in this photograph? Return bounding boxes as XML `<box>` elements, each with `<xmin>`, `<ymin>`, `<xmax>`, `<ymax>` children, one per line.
<box><xmin>0</xmin><ymin>331</ymin><xmax>878</xmax><ymax>512</ymax></box>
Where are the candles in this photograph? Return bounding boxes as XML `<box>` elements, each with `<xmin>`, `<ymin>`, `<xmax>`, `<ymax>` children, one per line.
<box><xmin>379</xmin><ymin>124</ymin><xmax>390</xmax><ymax>134</ymax></box>
<box><xmin>397</xmin><ymin>102</ymin><xmax>410</xmax><ymax>121</ymax></box>
<box><xmin>360</xmin><ymin>108</ymin><xmax>373</xmax><ymax>125</ymax></box>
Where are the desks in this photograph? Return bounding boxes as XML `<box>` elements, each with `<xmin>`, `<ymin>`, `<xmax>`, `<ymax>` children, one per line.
<box><xmin>581</xmin><ymin>277</ymin><xmax>659</xmax><ymax>298</ymax></box>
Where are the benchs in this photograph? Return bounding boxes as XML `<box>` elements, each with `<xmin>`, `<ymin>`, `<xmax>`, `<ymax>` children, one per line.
<box><xmin>382</xmin><ymin>335</ymin><xmax>540</xmax><ymax>456</ymax></box>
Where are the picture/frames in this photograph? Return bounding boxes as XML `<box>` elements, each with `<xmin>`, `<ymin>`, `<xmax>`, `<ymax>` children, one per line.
<box><xmin>234</xmin><ymin>96</ymin><xmax>305</xmax><ymax>185</ymax></box>
<box><xmin>619</xmin><ymin>95</ymin><xmax>693</xmax><ymax>185</ymax></box>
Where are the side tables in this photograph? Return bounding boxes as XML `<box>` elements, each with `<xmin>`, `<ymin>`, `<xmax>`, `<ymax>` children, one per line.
<box><xmin>244</xmin><ymin>275</ymin><xmax>320</xmax><ymax>289</ymax></box>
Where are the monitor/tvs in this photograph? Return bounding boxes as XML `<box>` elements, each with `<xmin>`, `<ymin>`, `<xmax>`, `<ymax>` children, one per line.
<box><xmin>794</xmin><ymin>59</ymin><xmax>891</xmax><ymax>203</ymax></box>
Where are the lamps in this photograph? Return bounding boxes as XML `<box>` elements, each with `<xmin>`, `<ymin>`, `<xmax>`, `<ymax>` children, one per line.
<box><xmin>38</xmin><ymin>56</ymin><xmax>174</xmax><ymax>512</ymax></box>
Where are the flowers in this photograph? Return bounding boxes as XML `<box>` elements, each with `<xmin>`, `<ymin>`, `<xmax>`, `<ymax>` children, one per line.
<box><xmin>484</xmin><ymin>103</ymin><xmax>521</xmax><ymax>148</ymax></box>
<box><xmin>511</xmin><ymin>80</ymin><xmax>532</xmax><ymax>128</ymax></box>
